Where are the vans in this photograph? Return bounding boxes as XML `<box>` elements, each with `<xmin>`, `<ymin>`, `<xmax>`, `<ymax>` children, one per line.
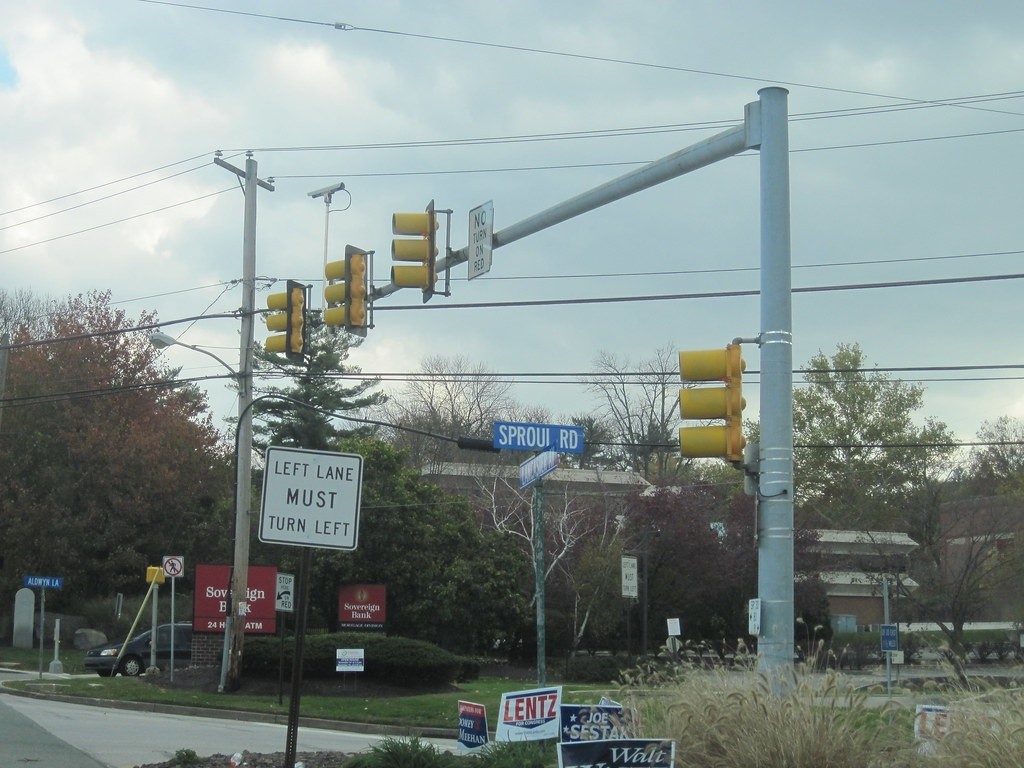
<box><xmin>84</xmin><ymin>620</ymin><xmax>194</xmax><ymax>677</ymax></box>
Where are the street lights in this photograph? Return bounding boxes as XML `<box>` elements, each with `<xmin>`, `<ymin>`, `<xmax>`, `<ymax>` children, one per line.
<box><xmin>149</xmin><ymin>332</ymin><xmax>251</xmax><ymax>678</ymax></box>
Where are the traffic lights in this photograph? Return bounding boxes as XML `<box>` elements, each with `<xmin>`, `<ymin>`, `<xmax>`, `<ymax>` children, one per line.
<box><xmin>326</xmin><ymin>245</ymin><xmax>368</xmax><ymax>338</ymax></box>
<box><xmin>390</xmin><ymin>212</ymin><xmax>439</xmax><ymax>291</ymax></box>
<box><xmin>678</xmin><ymin>344</ymin><xmax>746</xmax><ymax>460</ymax></box>
<box><xmin>267</xmin><ymin>281</ymin><xmax>304</xmax><ymax>360</ymax></box>
